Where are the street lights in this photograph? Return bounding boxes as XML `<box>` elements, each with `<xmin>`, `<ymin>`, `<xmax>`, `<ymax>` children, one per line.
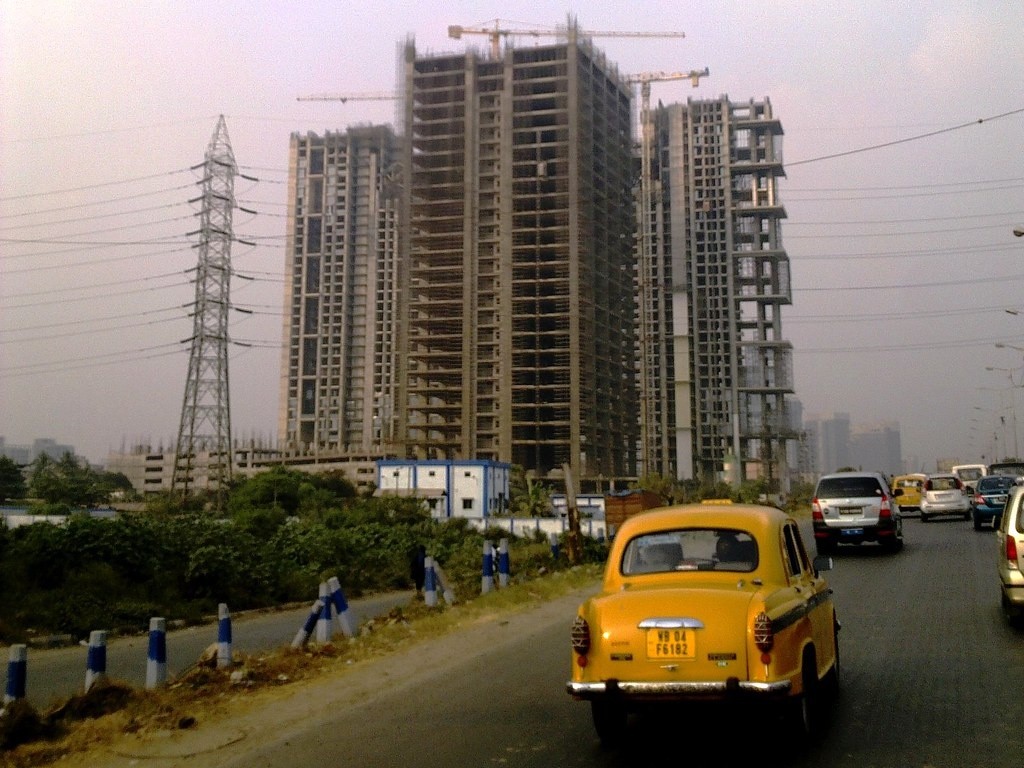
<box><xmin>968</xmin><ymin>366</ymin><xmax>1024</xmax><ymax>462</ymax></box>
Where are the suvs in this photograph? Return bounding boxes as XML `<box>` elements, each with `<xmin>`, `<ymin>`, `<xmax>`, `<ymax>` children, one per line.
<box><xmin>812</xmin><ymin>471</ymin><xmax>904</xmax><ymax>558</ymax></box>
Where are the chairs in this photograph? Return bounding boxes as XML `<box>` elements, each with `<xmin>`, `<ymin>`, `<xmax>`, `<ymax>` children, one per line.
<box><xmin>713</xmin><ymin>561</ymin><xmax>753</xmax><ymax>570</ymax></box>
<box><xmin>632</xmin><ymin>543</ymin><xmax>682</xmax><ymax>571</ymax></box>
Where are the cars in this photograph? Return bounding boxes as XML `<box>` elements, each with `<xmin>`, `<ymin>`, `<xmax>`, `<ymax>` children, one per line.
<box><xmin>893</xmin><ymin>475</ymin><xmax>926</xmax><ymax>513</ymax></box>
<box><xmin>916</xmin><ymin>475</ymin><xmax>972</xmax><ymax>523</ymax></box>
<box><xmin>992</xmin><ymin>484</ymin><xmax>1024</xmax><ymax>629</ymax></box>
<box><xmin>561</xmin><ymin>498</ymin><xmax>843</xmax><ymax>743</ymax></box>
<box><xmin>972</xmin><ymin>476</ymin><xmax>1018</xmax><ymax>532</ymax></box>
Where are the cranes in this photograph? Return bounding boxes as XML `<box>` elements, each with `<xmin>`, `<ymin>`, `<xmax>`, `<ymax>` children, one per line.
<box><xmin>448</xmin><ymin>16</ymin><xmax>686</xmax><ymax>66</ymax></box>
<box><xmin>623</xmin><ymin>66</ymin><xmax>710</xmax><ymax>477</ymax></box>
<box><xmin>293</xmin><ymin>91</ymin><xmax>402</xmax><ymax>105</ymax></box>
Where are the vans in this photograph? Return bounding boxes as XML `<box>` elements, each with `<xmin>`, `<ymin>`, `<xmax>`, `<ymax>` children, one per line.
<box><xmin>952</xmin><ymin>464</ymin><xmax>989</xmax><ymax>501</ymax></box>
<box><xmin>988</xmin><ymin>462</ymin><xmax>1024</xmax><ymax>486</ymax></box>
